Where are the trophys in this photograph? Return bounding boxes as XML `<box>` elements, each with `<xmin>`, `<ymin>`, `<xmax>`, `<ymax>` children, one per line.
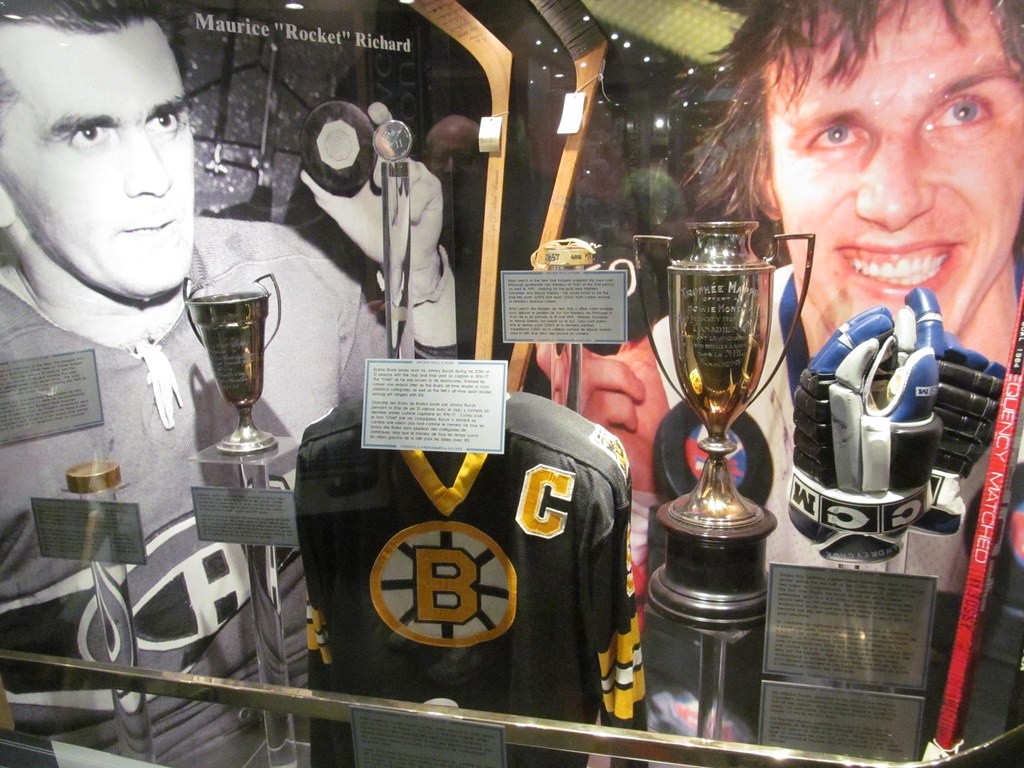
<box><xmin>635</xmin><ymin>218</ymin><xmax>814</xmax><ymax>631</ymax></box>
<box><xmin>180</xmin><ymin>272</ymin><xmax>297</xmax><ymax>452</ymax></box>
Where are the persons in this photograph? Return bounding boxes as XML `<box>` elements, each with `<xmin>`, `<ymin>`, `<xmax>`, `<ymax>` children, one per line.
<box><xmin>0</xmin><ymin>0</ymin><xmax>460</xmax><ymax>741</ymax></box>
<box><xmin>537</xmin><ymin>0</ymin><xmax>1024</xmax><ymax>599</ymax></box>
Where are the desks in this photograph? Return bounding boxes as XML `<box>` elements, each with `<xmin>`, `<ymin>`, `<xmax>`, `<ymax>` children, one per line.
<box><xmin>644</xmin><ymin>603</ymin><xmax>754</xmax><ymax>739</ymax></box>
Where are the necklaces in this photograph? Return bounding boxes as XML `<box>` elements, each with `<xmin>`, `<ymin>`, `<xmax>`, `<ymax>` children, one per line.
<box><xmin>81</xmin><ymin>316</ymin><xmax>185</xmax><ymax>428</ymax></box>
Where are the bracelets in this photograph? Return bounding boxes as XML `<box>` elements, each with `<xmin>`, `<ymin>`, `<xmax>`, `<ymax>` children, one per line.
<box><xmin>376</xmin><ymin>246</ymin><xmax>449</xmax><ymax>307</ymax></box>
